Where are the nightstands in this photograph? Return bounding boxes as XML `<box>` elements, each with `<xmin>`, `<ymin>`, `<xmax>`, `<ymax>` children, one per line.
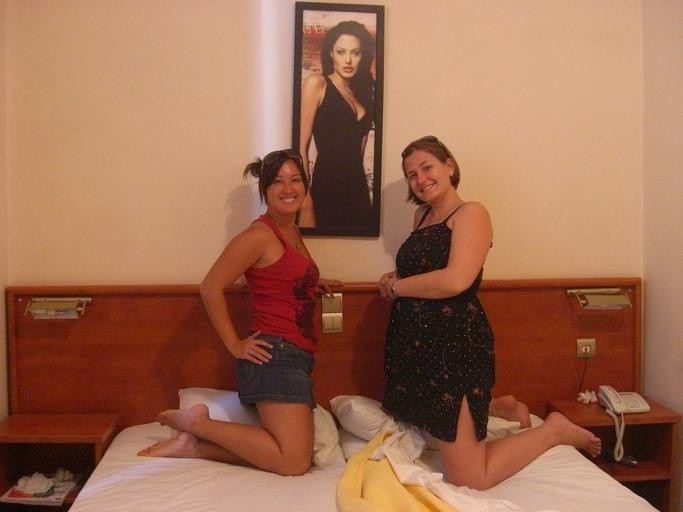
<box><xmin>545</xmin><ymin>394</ymin><xmax>683</xmax><ymax>512</ymax></box>
<box><xmin>0</xmin><ymin>413</ymin><xmax>120</xmax><ymax>512</ymax></box>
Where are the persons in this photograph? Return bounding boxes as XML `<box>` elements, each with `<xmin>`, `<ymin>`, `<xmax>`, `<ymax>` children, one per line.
<box><xmin>378</xmin><ymin>137</ymin><xmax>601</xmax><ymax>492</ymax></box>
<box><xmin>298</xmin><ymin>20</ymin><xmax>375</xmax><ymax>230</ymax></box>
<box><xmin>137</xmin><ymin>148</ymin><xmax>345</xmax><ymax>477</ymax></box>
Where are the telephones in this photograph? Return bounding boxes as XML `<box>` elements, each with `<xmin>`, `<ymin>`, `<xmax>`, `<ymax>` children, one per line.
<box><xmin>598</xmin><ymin>385</ymin><xmax>651</xmax><ymax>415</ymax></box>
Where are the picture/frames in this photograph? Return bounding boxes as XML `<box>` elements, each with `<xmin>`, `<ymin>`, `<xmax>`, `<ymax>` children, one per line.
<box><xmin>292</xmin><ymin>1</ymin><xmax>385</xmax><ymax>237</ymax></box>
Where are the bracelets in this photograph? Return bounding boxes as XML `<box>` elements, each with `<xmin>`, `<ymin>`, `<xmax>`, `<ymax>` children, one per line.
<box><xmin>390</xmin><ymin>281</ymin><xmax>398</xmax><ymax>299</ymax></box>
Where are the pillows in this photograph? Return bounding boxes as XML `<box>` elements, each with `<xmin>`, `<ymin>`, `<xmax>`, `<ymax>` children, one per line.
<box><xmin>178</xmin><ymin>387</ymin><xmax>340</xmax><ymax>470</ymax></box>
<box><xmin>330</xmin><ymin>394</ymin><xmax>521</xmax><ymax>450</ymax></box>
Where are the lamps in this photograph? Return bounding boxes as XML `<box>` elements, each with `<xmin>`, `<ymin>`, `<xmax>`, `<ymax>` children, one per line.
<box><xmin>567</xmin><ymin>288</ymin><xmax>633</xmax><ymax>312</ymax></box>
<box><xmin>23</xmin><ymin>297</ymin><xmax>92</xmax><ymax>320</ymax></box>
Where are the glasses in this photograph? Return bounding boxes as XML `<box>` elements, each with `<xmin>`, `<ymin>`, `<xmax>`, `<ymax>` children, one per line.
<box><xmin>261</xmin><ymin>149</ymin><xmax>303</xmax><ymax>177</ymax></box>
<box><xmin>402</xmin><ymin>136</ymin><xmax>451</xmax><ymax>159</ymax></box>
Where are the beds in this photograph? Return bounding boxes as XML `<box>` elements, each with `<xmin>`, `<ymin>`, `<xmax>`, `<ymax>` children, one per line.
<box><xmin>67</xmin><ymin>420</ymin><xmax>348</xmax><ymax>512</ymax></box>
<box><xmin>336</xmin><ymin>412</ymin><xmax>660</xmax><ymax>512</ymax></box>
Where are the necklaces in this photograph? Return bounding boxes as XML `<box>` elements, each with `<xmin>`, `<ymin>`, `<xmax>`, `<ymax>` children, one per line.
<box><xmin>281</xmin><ymin>227</ymin><xmax>301</xmax><ymax>249</ymax></box>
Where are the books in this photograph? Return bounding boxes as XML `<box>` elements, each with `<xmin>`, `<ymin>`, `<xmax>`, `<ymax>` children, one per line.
<box><xmin>0</xmin><ymin>480</ymin><xmax>76</xmax><ymax>507</ymax></box>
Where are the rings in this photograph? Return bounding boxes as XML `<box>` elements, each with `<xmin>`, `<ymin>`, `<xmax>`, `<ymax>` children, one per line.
<box><xmin>251</xmin><ymin>350</ymin><xmax>255</xmax><ymax>354</ymax></box>
